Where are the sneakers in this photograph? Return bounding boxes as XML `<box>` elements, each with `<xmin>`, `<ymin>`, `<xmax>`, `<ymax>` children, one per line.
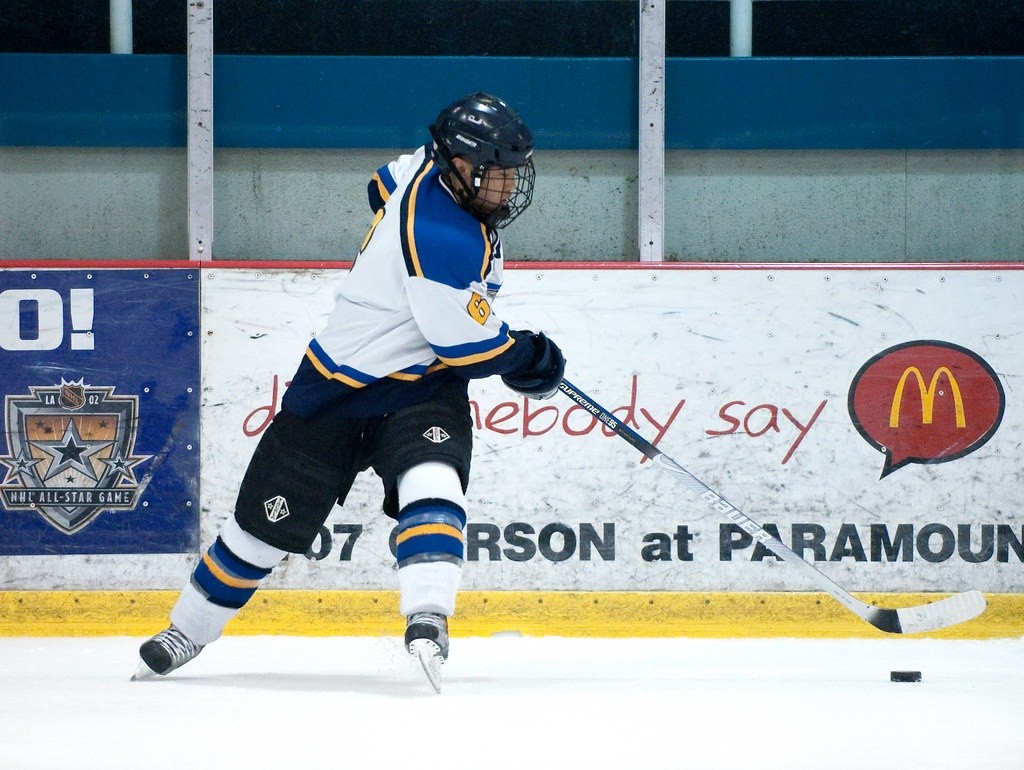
<box><xmin>405</xmin><ymin>610</ymin><xmax>450</xmax><ymax>693</ymax></box>
<box><xmin>131</xmin><ymin>622</ymin><xmax>206</xmax><ymax>681</ymax></box>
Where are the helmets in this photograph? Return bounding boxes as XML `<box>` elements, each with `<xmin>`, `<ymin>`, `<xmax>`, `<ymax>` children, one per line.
<box><xmin>429</xmin><ymin>92</ymin><xmax>537</xmax><ymax>229</ymax></box>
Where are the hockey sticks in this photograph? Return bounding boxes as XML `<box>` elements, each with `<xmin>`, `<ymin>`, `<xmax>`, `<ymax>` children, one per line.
<box><xmin>555</xmin><ymin>376</ymin><xmax>989</xmax><ymax>635</ymax></box>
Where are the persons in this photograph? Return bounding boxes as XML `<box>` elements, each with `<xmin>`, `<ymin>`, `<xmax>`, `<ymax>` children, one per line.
<box><xmin>130</xmin><ymin>93</ymin><xmax>566</xmax><ymax>697</ymax></box>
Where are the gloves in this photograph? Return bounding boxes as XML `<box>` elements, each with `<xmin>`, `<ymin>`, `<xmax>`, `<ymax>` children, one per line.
<box><xmin>501</xmin><ymin>330</ymin><xmax>567</xmax><ymax>401</ymax></box>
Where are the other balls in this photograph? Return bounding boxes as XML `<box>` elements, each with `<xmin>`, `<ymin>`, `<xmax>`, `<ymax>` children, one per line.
<box><xmin>891</xmin><ymin>670</ymin><xmax>923</xmax><ymax>683</ymax></box>
